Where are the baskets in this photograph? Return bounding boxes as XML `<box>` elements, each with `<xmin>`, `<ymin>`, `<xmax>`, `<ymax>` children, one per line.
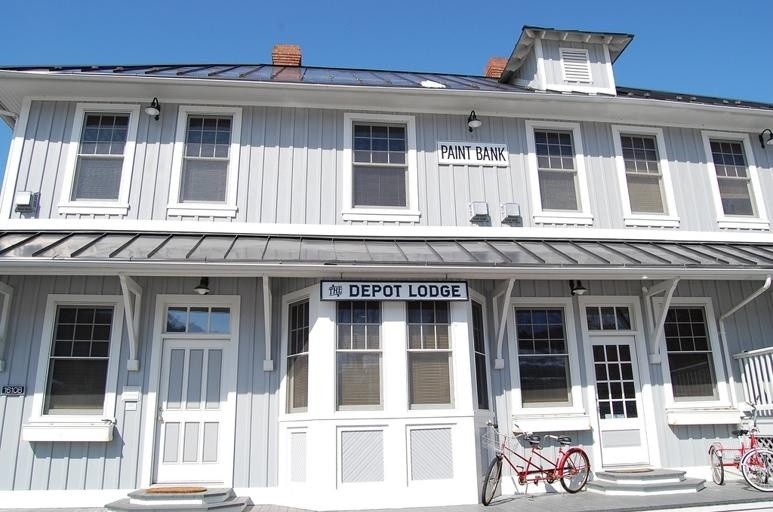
<box><xmin>481</xmin><ymin>432</ymin><xmax>517</xmax><ymax>454</ymax></box>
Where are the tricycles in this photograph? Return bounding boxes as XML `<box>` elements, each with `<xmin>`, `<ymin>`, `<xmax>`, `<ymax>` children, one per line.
<box><xmin>706</xmin><ymin>394</ymin><xmax>773</xmax><ymax>495</ymax></box>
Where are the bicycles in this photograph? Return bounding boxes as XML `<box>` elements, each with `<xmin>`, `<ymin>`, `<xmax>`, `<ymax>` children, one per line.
<box><xmin>481</xmin><ymin>418</ymin><xmax>591</xmax><ymax>507</ymax></box>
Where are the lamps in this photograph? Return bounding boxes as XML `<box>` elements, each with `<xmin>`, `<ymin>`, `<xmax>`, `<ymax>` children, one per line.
<box><xmin>758</xmin><ymin>128</ymin><xmax>773</xmax><ymax>148</ymax></box>
<box><xmin>194</xmin><ymin>277</ymin><xmax>210</xmax><ymax>295</ymax></box>
<box><xmin>568</xmin><ymin>280</ymin><xmax>587</xmax><ymax>295</ymax></box>
<box><xmin>144</xmin><ymin>96</ymin><xmax>162</xmax><ymax>121</ymax></box>
<box><xmin>466</xmin><ymin>111</ymin><xmax>482</xmax><ymax>132</ymax></box>
